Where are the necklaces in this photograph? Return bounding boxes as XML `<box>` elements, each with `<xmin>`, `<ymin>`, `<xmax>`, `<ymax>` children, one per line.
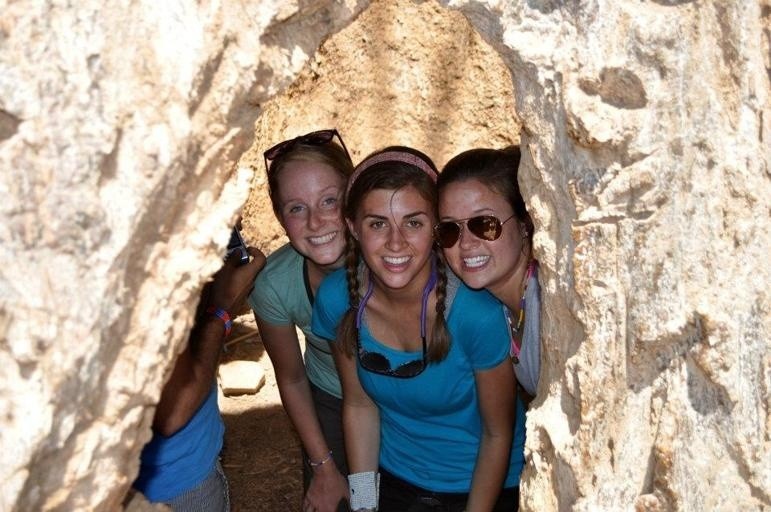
<box><xmin>508</xmin><ymin>264</ymin><xmax>533</xmax><ymax>364</ymax></box>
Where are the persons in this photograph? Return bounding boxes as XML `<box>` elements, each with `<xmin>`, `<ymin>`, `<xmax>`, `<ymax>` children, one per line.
<box><xmin>312</xmin><ymin>146</ymin><xmax>528</xmax><ymax>512</ymax></box>
<box><xmin>432</xmin><ymin>146</ymin><xmax>541</xmax><ymax>399</ymax></box>
<box><xmin>131</xmin><ymin>216</ymin><xmax>267</xmax><ymax>512</ymax></box>
<box><xmin>245</xmin><ymin>135</ymin><xmax>355</xmax><ymax>512</ymax></box>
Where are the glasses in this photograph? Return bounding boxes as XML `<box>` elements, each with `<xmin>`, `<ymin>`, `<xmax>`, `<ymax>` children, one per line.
<box><xmin>356</xmin><ymin>328</ymin><xmax>428</xmax><ymax>378</ymax></box>
<box><xmin>264</xmin><ymin>130</ymin><xmax>353</xmax><ymax>175</ymax></box>
<box><xmin>433</xmin><ymin>215</ymin><xmax>514</xmax><ymax>248</ymax></box>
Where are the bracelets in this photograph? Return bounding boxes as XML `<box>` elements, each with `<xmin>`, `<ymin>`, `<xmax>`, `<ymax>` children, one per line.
<box><xmin>347</xmin><ymin>472</ymin><xmax>381</xmax><ymax>511</ymax></box>
<box><xmin>312</xmin><ymin>453</ymin><xmax>333</xmax><ymax>469</ymax></box>
<box><xmin>212</xmin><ymin>305</ymin><xmax>232</xmax><ymax>336</ymax></box>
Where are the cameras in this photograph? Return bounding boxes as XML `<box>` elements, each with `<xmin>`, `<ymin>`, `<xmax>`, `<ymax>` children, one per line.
<box><xmin>224</xmin><ymin>224</ymin><xmax>249</xmax><ymax>267</ymax></box>
